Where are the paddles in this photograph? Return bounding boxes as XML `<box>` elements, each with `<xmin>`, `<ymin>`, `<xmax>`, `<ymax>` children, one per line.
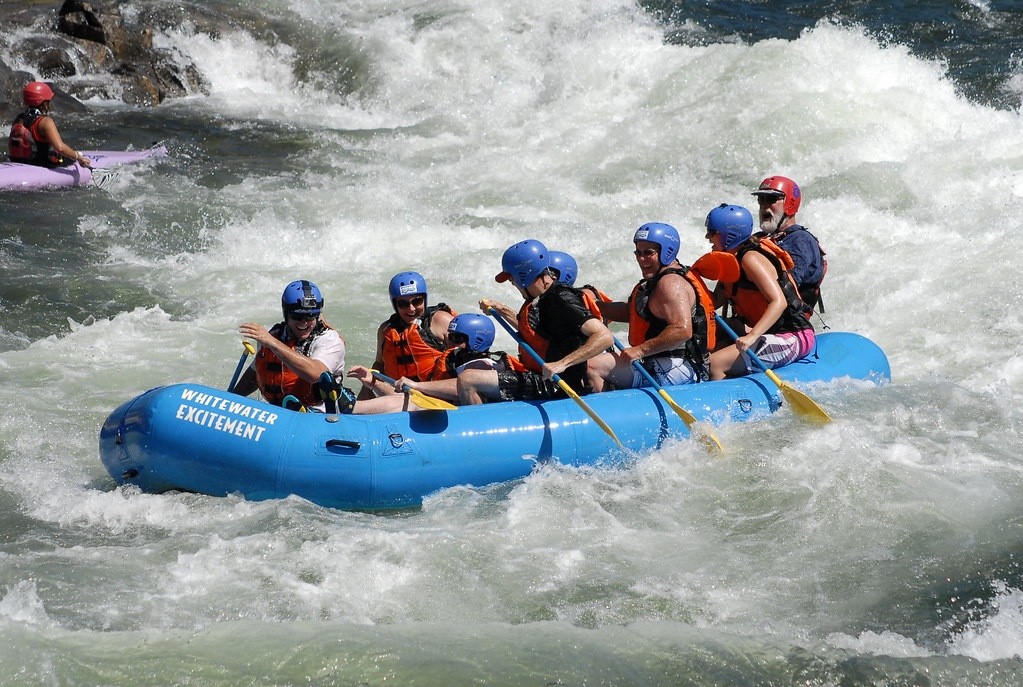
<box><xmin>713</xmin><ymin>311</ymin><xmax>831</xmax><ymax>423</ymax></box>
<box><xmin>369</xmin><ymin>369</ymin><xmax>458</xmax><ymax>410</ymax></box>
<box><xmin>228</xmin><ymin>341</ymin><xmax>255</xmax><ymax>392</ymax></box>
<box><xmin>613</xmin><ymin>335</ymin><xmax>697</xmax><ymax>430</ymax></box>
<box><xmin>478</xmin><ymin>299</ymin><xmax>620</xmax><ymax>445</ymax></box>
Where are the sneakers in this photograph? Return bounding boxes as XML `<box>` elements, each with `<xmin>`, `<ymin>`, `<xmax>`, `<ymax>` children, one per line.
<box><xmin>318</xmin><ymin>371</ymin><xmax>357</xmax><ymax>414</ymax></box>
<box><xmin>281</xmin><ymin>395</ymin><xmax>324</xmax><ymax>413</ymax></box>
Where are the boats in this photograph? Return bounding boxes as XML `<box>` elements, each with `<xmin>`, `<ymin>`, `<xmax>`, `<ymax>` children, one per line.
<box><xmin>0</xmin><ymin>145</ymin><xmax>168</xmax><ymax>189</ymax></box>
<box><xmin>99</xmin><ymin>331</ymin><xmax>889</xmax><ymax>510</ymax></box>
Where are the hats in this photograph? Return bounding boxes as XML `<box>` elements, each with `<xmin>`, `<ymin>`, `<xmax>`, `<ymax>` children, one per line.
<box><xmin>750</xmin><ymin>188</ymin><xmax>784</xmax><ymax>196</ymax></box>
<box><xmin>495</xmin><ymin>271</ymin><xmax>512</xmax><ymax>283</ymax></box>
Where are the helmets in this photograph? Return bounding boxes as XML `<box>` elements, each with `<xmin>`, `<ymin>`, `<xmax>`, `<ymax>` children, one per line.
<box><xmin>446</xmin><ymin>312</ymin><xmax>496</xmax><ymax>357</ymax></box>
<box><xmin>704</xmin><ymin>202</ymin><xmax>754</xmax><ymax>251</ymax></box>
<box><xmin>547</xmin><ymin>250</ymin><xmax>578</xmax><ymax>288</ymax></box>
<box><xmin>387</xmin><ymin>271</ymin><xmax>428</xmax><ymax>314</ymax></box>
<box><xmin>23</xmin><ymin>81</ymin><xmax>55</xmax><ymax>107</ymax></box>
<box><xmin>501</xmin><ymin>238</ymin><xmax>551</xmax><ymax>289</ymax></box>
<box><xmin>633</xmin><ymin>222</ymin><xmax>681</xmax><ymax>267</ymax></box>
<box><xmin>281</xmin><ymin>279</ymin><xmax>324</xmax><ymax>322</ymax></box>
<box><xmin>758</xmin><ymin>175</ymin><xmax>802</xmax><ymax>217</ymax></box>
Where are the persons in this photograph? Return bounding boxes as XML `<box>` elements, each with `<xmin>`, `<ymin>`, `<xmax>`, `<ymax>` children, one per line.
<box><xmin>320</xmin><ymin>238</ymin><xmax>616</xmax><ymax>417</ymax></box>
<box><xmin>234</xmin><ymin>279</ymin><xmax>348</xmax><ymax>413</ymax></box>
<box><xmin>750</xmin><ymin>176</ymin><xmax>828</xmax><ymax>321</ymax></box>
<box><xmin>585</xmin><ymin>222</ymin><xmax>716</xmax><ymax>393</ymax></box>
<box><xmin>6</xmin><ymin>83</ymin><xmax>91</xmax><ymax>170</ymax></box>
<box><xmin>704</xmin><ymin>203</ymin><xmax>815</xmax><ymax>382</ymax></box>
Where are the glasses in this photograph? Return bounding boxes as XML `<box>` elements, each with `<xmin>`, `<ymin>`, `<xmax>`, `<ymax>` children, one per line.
<box><xmin>757</xmin><ymin>194</ymin><xmax>784</xmax><ymax>205</ymax></box>
<box><xmin>633</xmin><ymin>247</ymin><xmax>661</xmax><ymax>257</ymax></box>
<box><xmin>706</xmin><ymin>227</ymin><xmax>720</xmax><ymax>238</ymax></box>
<box><xmin>447</xmin><ymin>332</ymin><xmax>469</xmax><ymax>344</ymax></box>
<box><xmin>285</xmin><ymin>311</ymin><xmax>318</xmax><ymax>322</ymax></box>
<box><xmin>393</xmin><ymin>297</ymin><xmax>425</xmax><ymax>310</ymax></box>
